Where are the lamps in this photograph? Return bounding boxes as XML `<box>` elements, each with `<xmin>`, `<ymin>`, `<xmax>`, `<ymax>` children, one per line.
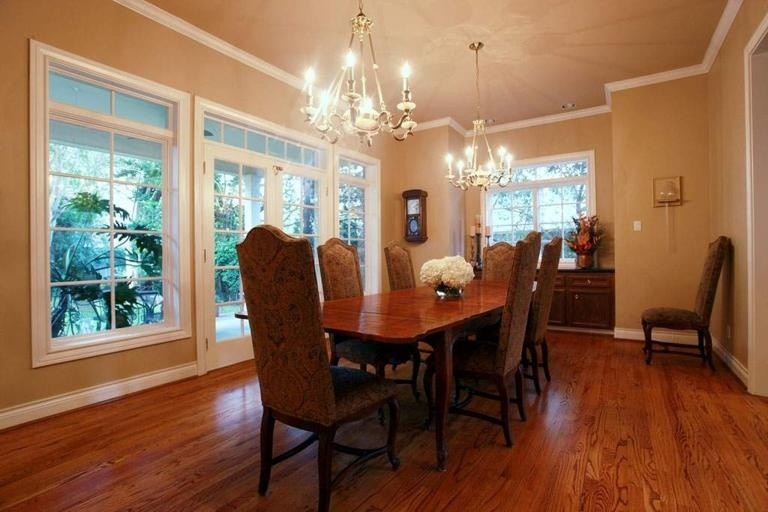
<box><xmin>445</xmin><ymin>42</ymin><xmax>514</xmax><ymax>192</ymax></box>
<box><xmin>298</xmin><ymin>1</ymin><xmax>417</xmax><ymax>148</ymax></box>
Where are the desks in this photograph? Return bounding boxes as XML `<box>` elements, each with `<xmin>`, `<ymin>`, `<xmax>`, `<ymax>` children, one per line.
<box><xmin>235</xmin><ymin>279</ymin><xmax>537</xmax><ymax>472</ymax></box>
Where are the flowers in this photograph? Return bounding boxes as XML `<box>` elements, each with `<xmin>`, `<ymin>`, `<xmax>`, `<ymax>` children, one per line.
<box><xmin>565</xmin><ymin>211</ymin><xmax>606</xmax><ymax>255</ymax></box>
<box><xmin>420</xmin><ymin>255</ymin><xmax>476</xmax><ymax>289</ymax></box>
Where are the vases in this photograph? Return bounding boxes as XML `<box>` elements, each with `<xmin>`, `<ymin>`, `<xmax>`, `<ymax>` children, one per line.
<box><xmin>435</xmin><ymin>282</ymin><xmax>464</xmax><ymax>299</ymax></box>
<box><xmin>577</xmin><ymin>253</ymin><xmax>594</xmax><ymax>270</ymax></box>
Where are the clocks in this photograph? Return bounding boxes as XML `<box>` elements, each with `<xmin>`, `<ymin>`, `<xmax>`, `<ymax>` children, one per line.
<box><xmin>402</xmin><ymin>189</ymin><xmax>428</xmax><ymax>243</ymax></box>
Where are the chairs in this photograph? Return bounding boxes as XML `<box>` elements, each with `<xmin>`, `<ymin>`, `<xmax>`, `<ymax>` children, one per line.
<box><xmin>384</xmin><ymin>240</ymin><xmax>438</xmax><ymax>370</ymax></box>
<box><xmin>641</xmin><ymin>236</ymin><xmax>727</xmax><ymax>370</ymax></box>
<box><xmin>481</xmin><ymin>241</ymin><xmax>516</xmax><ymax>283</ymax></box>
<box><xmin>234</xmin><ymin>224</ymin><xmax>401</xmax><ymax>512</ymax></box>
<box><xmin>420</xmin><ymin>232</ymin><xmax>543</xmax><ymax>448</ymax></box>
<box><xmin>480</xmin><ymin>237</ymin><xmax>562</xmax><ymax>396</ymax></box>
<box><xmin>316</xmin><ymin>238</ymin><xmax>423</xmax><ymax>427</ymax></box>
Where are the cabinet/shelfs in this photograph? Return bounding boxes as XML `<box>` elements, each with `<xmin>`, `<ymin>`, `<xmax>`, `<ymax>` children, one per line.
<box><xmin>567</xmin><ymin>271</ymin><xmax>615</xmax><ymax>336</ymax></box>
<box><xmin>531</xmin><ymin>271</ymin><xmax>567</xmax><ymax>334</ymax></box>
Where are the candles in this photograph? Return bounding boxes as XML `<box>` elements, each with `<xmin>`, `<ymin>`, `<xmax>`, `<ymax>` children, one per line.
<box><xmin>476</xmin><ymin>215</ymin><xmax>481</xmax><ymax>234</ymax></box>
<box><xmin>470</xmin><ymin>226</ymin><xmax>475</xmax><ymax>237</ymax></box>
<box><xmin>485</xmin><ymin>226</ymin><xmax>490</xmax><ymax>235</ymax></box>
